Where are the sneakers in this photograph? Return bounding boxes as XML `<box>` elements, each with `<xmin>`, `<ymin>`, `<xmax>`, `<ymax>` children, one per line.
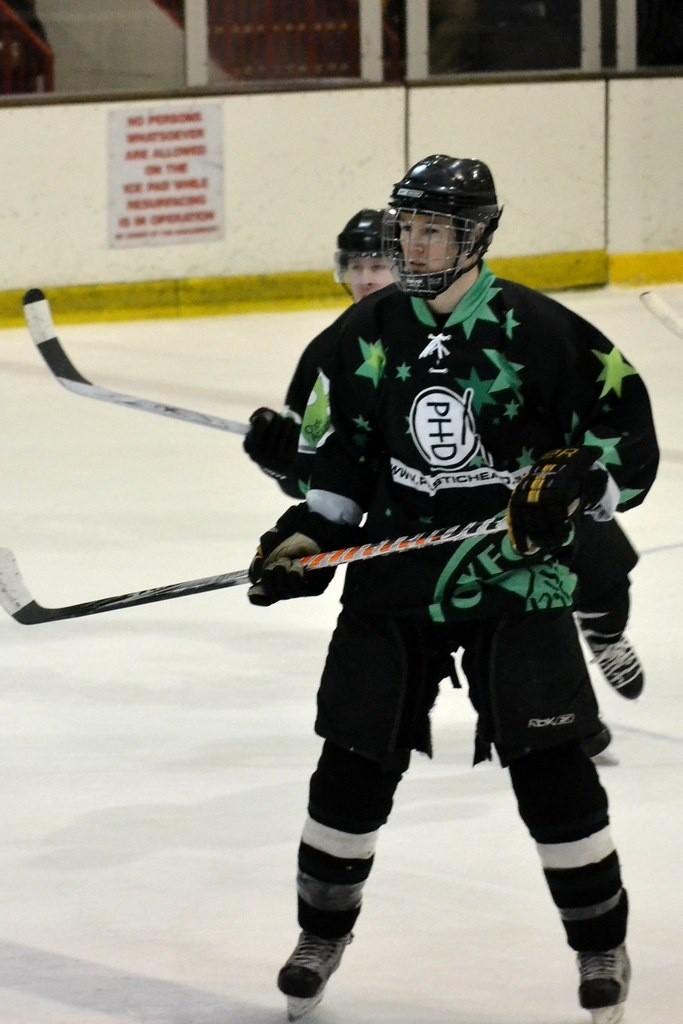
<box><xmin>587</xmin><ymin>636</ymin><xmax>645</xmax><ymax>699</ymax></box>
<box><xmin>277</xmin><ymin>931</ymin><xmax>354</xmax><ymax>1022</ymax></box>
<box><xmin>576</xmin><ymin>940</ymin><xmax>631</xmax><ymax>1024</ymax></box>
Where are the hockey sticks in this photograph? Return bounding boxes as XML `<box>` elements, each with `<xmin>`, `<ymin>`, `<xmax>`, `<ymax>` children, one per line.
<box><xmin>23</xmin><ymin>288</ymin><xmax>252</xmax><ymax>435</ymax></box>
<box><xmin>0</xmin><ymin>498</ymin><xmax>584</xmax><ymax>625</ymax></box>
<box><xmin>638</xmin><ymin>290</ymin><xmax>683</xmax><ymax>341</ymax></box>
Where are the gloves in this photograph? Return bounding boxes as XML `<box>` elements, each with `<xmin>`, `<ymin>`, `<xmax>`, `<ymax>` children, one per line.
<box><xmin>507</xmin><ymin>445</ymin><xmax>608</xmax><ymax>555</ymax></box>
<box><xmin>247</xmin><ymin>501</ymin><xmax>355</xmax><ymax>607</ymax></box>
<box><xmin>243</xmin><ymin>407</ymin><xmax>309</xmax><ymax>484</ymax></box>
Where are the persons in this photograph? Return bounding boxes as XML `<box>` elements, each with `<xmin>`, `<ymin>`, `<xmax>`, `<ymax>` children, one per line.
<box><xmin>243</xmin><ymin>207</ymin><xmax>646</xmax><ymax>768</ymax></box>
<box><xmin>0</xmin><ymin>18</ymin><xmax>50</xmax><ymax>93</ymax></box>
<box><xmin>248</xmin><ymin>150</ymin><xmax>661</xmax><ymax>1024</ymax></box>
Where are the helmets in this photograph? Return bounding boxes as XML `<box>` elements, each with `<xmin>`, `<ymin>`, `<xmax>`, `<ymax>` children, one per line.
<box><xmin>337</xmin><ymin>209</ymin><xmax>404</xmax><ymax>266</ymax></box>
<box><xmin>388</xmin><ymin>154</ymin><xmax>504</xmax><ymax>299</ymax></box>
